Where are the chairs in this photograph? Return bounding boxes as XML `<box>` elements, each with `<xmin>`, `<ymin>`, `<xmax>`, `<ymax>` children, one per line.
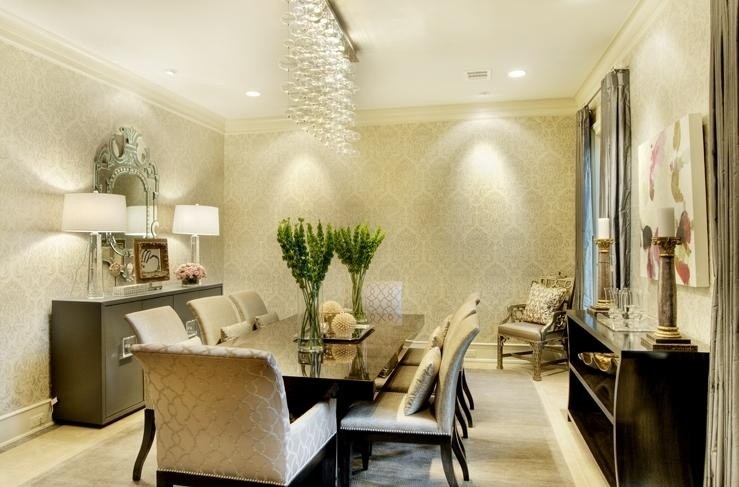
<box><xmin>187</xmin><ymin>290</ymin><xmax>279</xmax><ymax>347</ymax></box>
<box><xmin>124</xmin><ymin>306</ymin><xmax>188</xmax><ymax>482</ymax></box>
<box><xmin>496</xmin><ymin>270</ymin><xmax>577</xmax><ymax>381</ymax></box>
<box><xmin>338</xmin><ymin>312</ymin><xmax>480</xmax><ymax>487</ymax></box>
<box><xmin>365</xmin><ymin>281</ymin><xmax>402</xmax><ymax>314</ymax></box>
<box><xmin>375</xmin><ymin>291</ymin><xmax>482</xmax><ymax>440</ymax></box>
<box><xmin>129</xmin><ymin>343</ymin><xmax>337</xmax><ymax>487</ymax></box>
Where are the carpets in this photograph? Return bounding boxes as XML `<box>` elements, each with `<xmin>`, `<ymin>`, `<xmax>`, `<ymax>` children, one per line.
<box><xmin>21</xmin><ymin>366</ymin><xmax>575</xmax><ymax>487</ymax></box>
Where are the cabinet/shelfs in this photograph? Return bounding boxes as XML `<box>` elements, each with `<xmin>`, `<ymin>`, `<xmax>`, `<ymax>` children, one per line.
<box><xmin>50</xmin><ymin>282</ymin><xmax>225</xmax><ymax>428</ymax></box>
<box><xmin>563</xmin><ymin>307</ymin><xmax>712</xmax><ymax>487</ymax></box>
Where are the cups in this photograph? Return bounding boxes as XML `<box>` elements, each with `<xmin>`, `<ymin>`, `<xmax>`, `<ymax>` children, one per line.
<box><xmin>603</xmin><ymin>286</ymin><xmax>645</xmax><ymax>330</ymax></box>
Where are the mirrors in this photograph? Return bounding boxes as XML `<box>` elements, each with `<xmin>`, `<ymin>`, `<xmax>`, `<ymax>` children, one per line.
<box><xmin>87</xmin><ymin>126</ymin><xmax>160</xmax><ymax>296</ymax></box>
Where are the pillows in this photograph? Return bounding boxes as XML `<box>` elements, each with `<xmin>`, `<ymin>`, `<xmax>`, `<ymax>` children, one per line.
<box><xmin>522</xmin><ymin>280</ymin><xmax>568</xmax><ymax>325</ymax></box>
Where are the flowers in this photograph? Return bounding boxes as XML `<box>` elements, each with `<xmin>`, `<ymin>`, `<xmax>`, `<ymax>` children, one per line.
<box><xmin>175</xmin><ymin>263</ymin><xmax>207</xmax><ymax>281</ymax></box>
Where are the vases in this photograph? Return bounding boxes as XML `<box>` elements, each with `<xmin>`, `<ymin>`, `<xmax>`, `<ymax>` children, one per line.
<box><xmin>181</xmin><ymin>274</ymin><xmax>201</xmax><ymax>286</ymax></box>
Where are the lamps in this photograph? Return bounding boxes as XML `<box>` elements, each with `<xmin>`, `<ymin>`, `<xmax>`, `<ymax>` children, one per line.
<box><xmin>284</xmin><ymin>0</ymin><xmax>362</xmax><ymax>151</ymax></box>
<box><xmin>171</xmin><ymin>203</ymin><xmax>221</xmax><ymax>263</ymax></box>
<box><xmin>125</xmin><ymin>204</ymin><xmax>157</xmax><ymax>236</ymax></box>
<box><xmin>63</xmin><ymin>191</ymin><xmax>129</xmax><ymax>300</ymax></box>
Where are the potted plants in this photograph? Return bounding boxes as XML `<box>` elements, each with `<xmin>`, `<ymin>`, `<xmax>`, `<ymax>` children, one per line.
<box><xmin>332</xmin><ymin>221</ymin><xmax>386</xmax><ymax>324</ymax></box>
<box><xmin>276</xmin><ymin>215</ymin><xmax>337</xmax><ymax>353</ymax></box>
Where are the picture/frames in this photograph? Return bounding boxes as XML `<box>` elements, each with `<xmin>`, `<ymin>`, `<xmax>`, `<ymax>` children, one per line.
<box><xmin>101</xmin><ymin>237</ymin><xmax>127</xmax><ymax>283</ymax></box>
<box><xmin>133</xmin><ymin>237</ymin><xmax>170</xmax><ymax>283</ymax></box>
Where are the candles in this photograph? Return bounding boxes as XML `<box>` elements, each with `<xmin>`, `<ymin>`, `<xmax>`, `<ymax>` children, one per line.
<box><xmin>595</xmin><ymin>217</ymin><xmax>612</xmax><ymax>240</ymax></box>
<box><xmin>657</xmin><ymin>203</ymin><xmax>676</xmax><ymax>237</ymax></box>
